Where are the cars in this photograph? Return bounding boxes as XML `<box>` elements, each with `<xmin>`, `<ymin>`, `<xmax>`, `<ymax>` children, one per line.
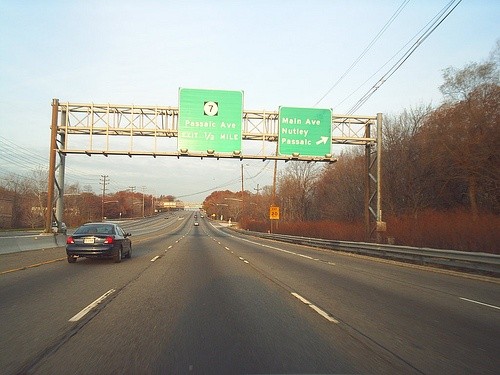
<box><xmin>66</xmin><ymin>222</ymin><xmax>132</xmax><ymax>263</ymax></box>
<box><xmin>52</xmin><ymin>221</ymin><xmax>67</xmax><ymax>233</ymax></box>
